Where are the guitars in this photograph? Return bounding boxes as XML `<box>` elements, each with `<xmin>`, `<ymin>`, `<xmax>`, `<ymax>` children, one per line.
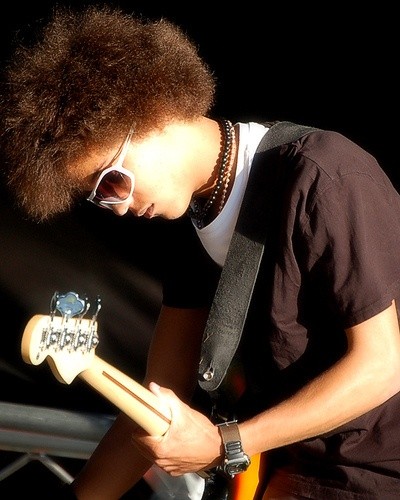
<box><xmin>21</xmin><ymin>293</ymin><xmax>275</xmax><ymax>500</ymax></box>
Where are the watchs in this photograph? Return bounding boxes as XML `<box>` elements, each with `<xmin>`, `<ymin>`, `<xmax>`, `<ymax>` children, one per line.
<box><xmin>216</xmin><ymin>418</ymin><xmax>251</xmax><ymax>478</ymax></box>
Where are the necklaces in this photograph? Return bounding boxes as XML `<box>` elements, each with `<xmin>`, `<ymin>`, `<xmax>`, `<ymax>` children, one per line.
<box><xmin>188</xmin><ymin>119</ymin><xmax>237</xmax><ymax>229</ymax></box>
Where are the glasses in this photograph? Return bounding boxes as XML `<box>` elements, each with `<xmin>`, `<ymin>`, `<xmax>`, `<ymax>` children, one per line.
<box><xmin>85</xmin><ymin>122</ymin><xmax>135</xmax><ymax>208</ymax></box>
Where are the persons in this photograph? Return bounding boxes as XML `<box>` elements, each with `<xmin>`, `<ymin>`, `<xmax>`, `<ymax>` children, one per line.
<box><xmin>0</xmin><ymin>11</ymin><xmax>400</xmax><ymax>500</ymax></box>
